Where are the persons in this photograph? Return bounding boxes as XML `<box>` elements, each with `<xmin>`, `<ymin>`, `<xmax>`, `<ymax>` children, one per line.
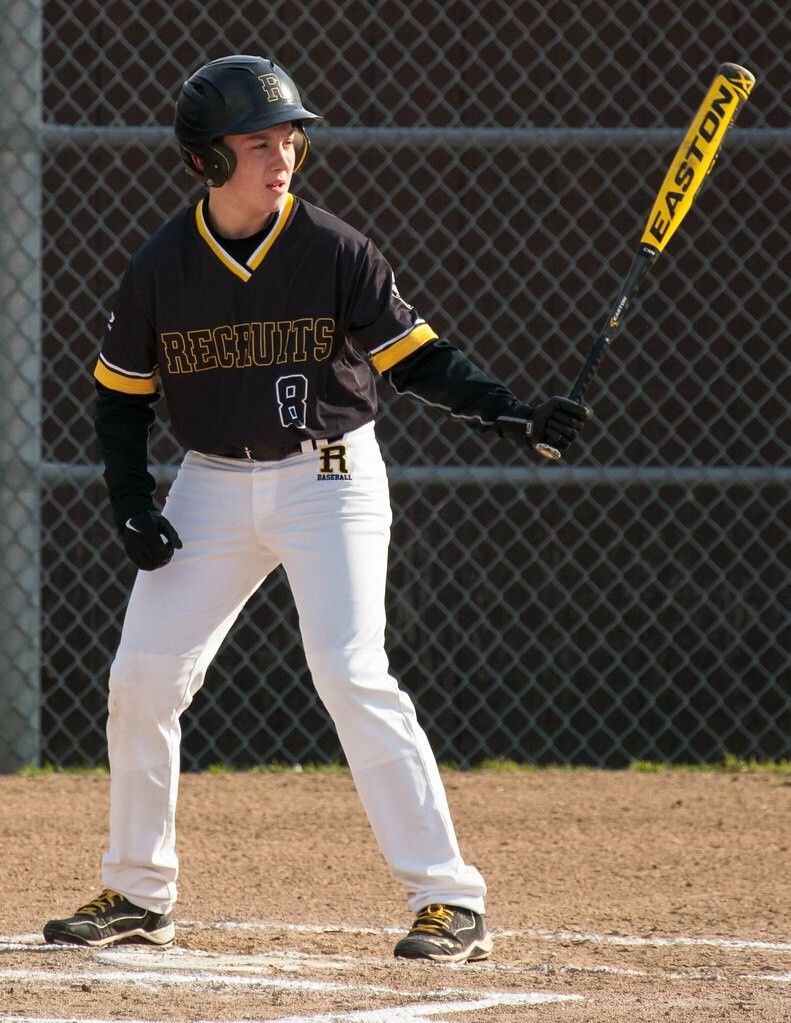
<box><xmin>43</xmin><ymin>52</ymin><xmax>592</xmax><ymax>967</ymax></box>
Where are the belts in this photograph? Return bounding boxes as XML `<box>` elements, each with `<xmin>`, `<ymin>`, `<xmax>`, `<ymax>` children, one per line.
<box><xmin>219</xmin><ymin>434</ymin><xmax>345</xmax><ymax>461</ymax></box>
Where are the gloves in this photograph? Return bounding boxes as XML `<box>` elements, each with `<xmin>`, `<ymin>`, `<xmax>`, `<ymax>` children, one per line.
<box><xmin>123</xmin><ymin>510</ymin><xmax>182</xmax><ymax>571</ymax></box>
<box><xmin>524</xmin><ymin>395</ymin><xmax>593</xmax><ymax>462</ymax></box>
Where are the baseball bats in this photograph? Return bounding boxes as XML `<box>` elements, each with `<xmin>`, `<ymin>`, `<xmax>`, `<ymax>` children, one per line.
<box><xmin>534</xmin><ymin>59</ymin><xmax>759</xmax><ymax>461</ymax></box>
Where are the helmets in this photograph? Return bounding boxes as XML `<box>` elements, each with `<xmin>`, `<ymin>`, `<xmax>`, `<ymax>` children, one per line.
<box><xmin>176</xmin><ymin>55</ymin><xmax>323</xmax><ymax>188</ymax></box>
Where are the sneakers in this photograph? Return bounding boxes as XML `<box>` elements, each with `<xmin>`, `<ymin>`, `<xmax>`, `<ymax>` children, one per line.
<box><xmin>43</xmin><ymin>888</ymin><xmax>175</xmax><ymax>946</ymax></box>
<box><xmin>394</xmin><ymin>903</ymin><xmax>493</xmax><ymax>962</ymax></box>
